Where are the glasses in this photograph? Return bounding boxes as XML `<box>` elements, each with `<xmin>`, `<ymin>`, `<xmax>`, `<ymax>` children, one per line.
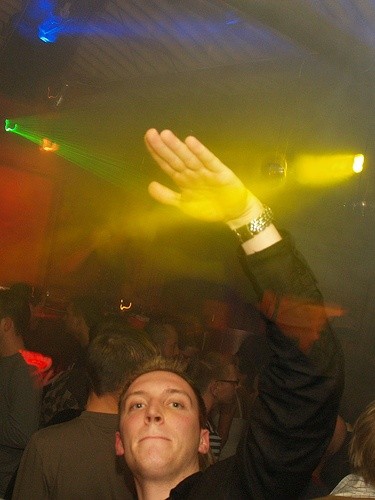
<box><xmin>216</xmin><ymin>379</ymin><xmax>240</xmax><ymax>386</ymax></box>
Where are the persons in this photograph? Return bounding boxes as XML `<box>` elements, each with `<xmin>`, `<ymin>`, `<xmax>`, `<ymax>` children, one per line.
<box><xmin>0</xmin><ymin>289</ymin><xmax>42</xmax><ymax>500</ymax></box>
<box><xmin>11</xmin><ymin>280</ymin><xmax>272</xmax><ymax>470</ymax></box>
<box><xmin>114</xmin><ymin>128</ymin><xmax>344</xmax><ymax>500</ymax></box>
<box><xmin>11</xmin><ymin>329</ymin><xmax>216</xmax><ymax>500</ymax></box>
<box><xmin>292</xmin><ymin>399</ymin><xmax>375</xmax><ymax>500</ymax></box>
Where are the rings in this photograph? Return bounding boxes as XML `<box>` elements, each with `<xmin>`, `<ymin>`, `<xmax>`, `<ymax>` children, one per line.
<box><xmin>178</xmin><ymin>165</ymin><xmax>190</xmax><ymax>174</ymax></box>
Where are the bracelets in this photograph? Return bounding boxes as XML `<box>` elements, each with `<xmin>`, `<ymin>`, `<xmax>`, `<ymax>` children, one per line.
<box><xmin>230</xmin><ymin>205</ymin><xmax>275</xmax><ymax>245</ymax></box>
